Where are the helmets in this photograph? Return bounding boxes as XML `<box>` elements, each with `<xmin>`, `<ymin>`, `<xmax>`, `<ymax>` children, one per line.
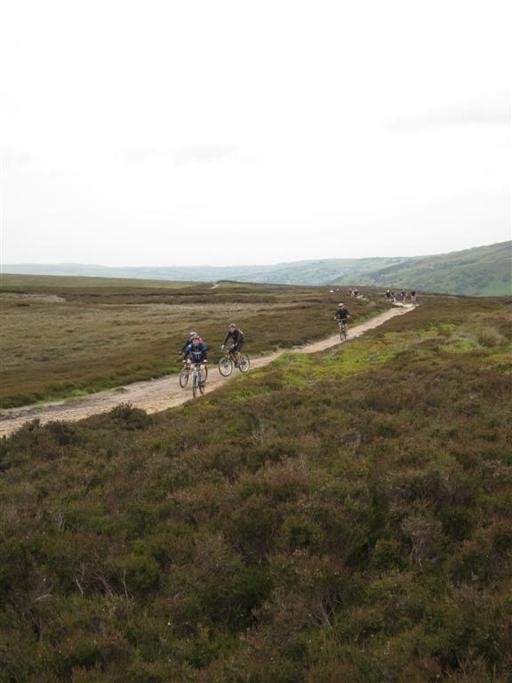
<box><xmin>338</xmin><ymin>303</ymin><xmax>344</xmax><ymax>307</ymax></box>
<box><xmin>190</xmin><ymin>332</ymin><xmax>199</xmax><ymax>340</ymax></box>
<box><xmin>229</xmin><ymin>323</ymin><xmax>236</xmax><ymax>327</ymax></box>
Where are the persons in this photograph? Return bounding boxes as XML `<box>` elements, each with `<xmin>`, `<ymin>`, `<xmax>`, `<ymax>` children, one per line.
<box><xmin>334</xmin><ymin>301</ymin><xmax>350</xmax><ymax>335</ymax></box>
<box><xmin>178</xmin><ymin>331</ymin><xmax>198</xmax><ymax>355</ymax></box>
<box><xmin>384</xmin><ymin>287</ymin><xmax>418</xmax><ymax>305</ymax></box>
<box><xmin>183</xmin><ymin>334</ymin><xmax>208</xmax><ymax>386</ymax></box>
<box><xmin>221</xmin><ymin>321</ymin><xmax>245</xmax><ymax>367</ymax></box>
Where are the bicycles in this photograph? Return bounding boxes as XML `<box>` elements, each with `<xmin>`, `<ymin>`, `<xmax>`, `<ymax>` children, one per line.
<box><xmin>335</xmin><ymin>318</ymin><xmax>349</xmax><ymax>342</ymax></box>
<box><xmin>386</xmin><ymin>295</ymin><xmax>418</xmax><ymax>309</ymax></box>
<box><xmin>218</xmin><ymin>343</ymin><xmax>250</xmax><ymax>376</ymax></box>
<box><xmin>187</xmin><ymin>361</ymin><xmax>209</xmax><ymax>399</ymax></box>
<box><xmin>179</xmin><ymin>353</ymin><xmax>209</xmax><ymax>387</ymax></box>
